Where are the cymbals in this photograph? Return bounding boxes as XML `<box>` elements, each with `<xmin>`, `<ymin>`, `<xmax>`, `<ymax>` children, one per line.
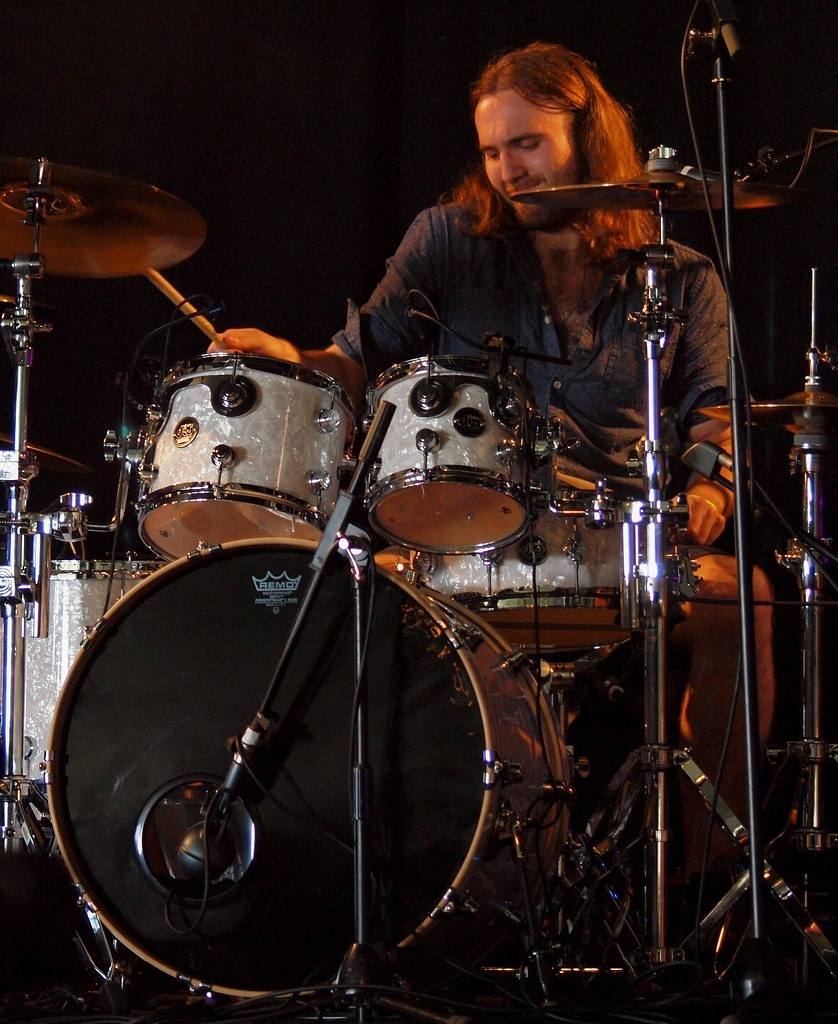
<box><xmin>509</xmin><ymin>171</ymin><xmax>797</xmax><ymax>209</ymax></box>
<box><xmin>697</xmin><ymin>390</ymin><xmax>838</xmax><ymax>435</ymax></box>
<box><xmin>0</xmin><ymin>152</ymin><xmax>206</xmax><ymax>278</ymax></box>
<box><xmin>0</xmin><ymin>433</ymin><xmax>94</xmax><ymax>476</ymax></box>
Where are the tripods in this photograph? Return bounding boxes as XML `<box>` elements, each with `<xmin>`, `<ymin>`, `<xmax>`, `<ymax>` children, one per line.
<box><xmin>518</xmin><ymin>255</ymin><xmax>838</xmax><ymax>990</ymax></box>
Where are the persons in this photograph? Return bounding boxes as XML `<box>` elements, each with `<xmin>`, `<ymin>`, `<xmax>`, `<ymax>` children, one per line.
<box><xmin>207</xmin><ymin>44</ymin><xmax>775</xmax><ymax>968</ymax></box>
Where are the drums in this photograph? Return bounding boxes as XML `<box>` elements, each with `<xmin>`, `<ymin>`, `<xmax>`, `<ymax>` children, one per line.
<box><xmin>364</xmin><ymin>356</ymin><xmax>534</xmax><ymax>556</ymax></box>
<box><xmin>413</xmin><ymin>495</ymin><xmax>690</xmax><ymax>653</ymax></box>
<box><xmin>45</xmin><ymin>536</ymin><xmax>567</xmax><ymax>998</ymax></box>
<box><xmin>20</xmin><ymin>559</ymin><xmax>172</xmax><ymax>840</ymax></box>
<box><xmin>138</xmin><ymin>353</ymin><xmax>354</xmax><ymax>565</ymax></box>
